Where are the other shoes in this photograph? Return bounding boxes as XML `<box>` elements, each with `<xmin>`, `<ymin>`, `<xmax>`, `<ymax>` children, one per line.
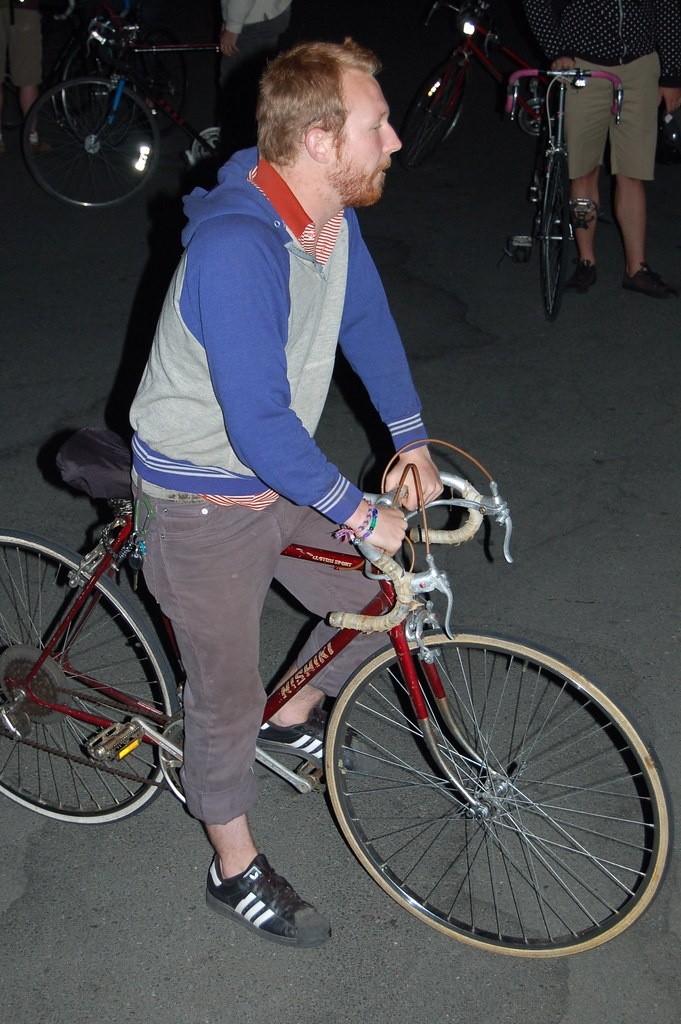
<box><xmin>0</xmin><ymin>140</ymin><xmax>6</xmax><ymax>152</ymax></box>
<box><xmin>29</xmin><ymin>141</ymin><xmax>54</xmax><ymax>151</ymax></box>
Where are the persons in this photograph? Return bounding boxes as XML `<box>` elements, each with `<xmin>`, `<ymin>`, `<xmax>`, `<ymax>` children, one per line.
<box><xmin>522</xmin><ymin>0</ymin><xmax>680</xmax><ymax>300</ymax></box>
<box><xmin>204</xmin><ymin>1</ymin><xmax>296</xmax><ymax>190</ymax></box>
<box><xmin>127</xmin><ymin>40</ymin><xmax>445</xmax><ymax>951</ymax></box>
<box><xmin>1</xmin><ymin>1</ymin><xmax>54</xmax><ymax>156</ymax></box>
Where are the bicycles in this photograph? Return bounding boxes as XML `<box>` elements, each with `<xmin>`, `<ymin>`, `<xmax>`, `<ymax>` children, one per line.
<box><xmin>503</xmin><ymin>68</ymin><xmax>624</xmax><ymax>322</ymax></box>
<box><xmin>398</xmin><ymin>0</ymin><xmax>565</xmax><ymax>169</ymax></box>
<box><xmin>22</xmin><ymin>11</ymin><xmax>277</xmax><ymax>209</ymax></box>
<box><xmin>0</xmin><ymin>429</ymin><xmax>674</xmax><ymax>959</ymax></box>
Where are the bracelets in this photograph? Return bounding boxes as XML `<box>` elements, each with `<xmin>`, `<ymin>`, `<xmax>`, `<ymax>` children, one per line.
<box><xmin>333</xmin><ymin>498</ymin><xmax>381</xmax><ymax>548</ymax></box>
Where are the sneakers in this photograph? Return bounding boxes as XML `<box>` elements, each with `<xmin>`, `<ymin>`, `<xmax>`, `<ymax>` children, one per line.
<box><xmin>563</xmin><ymin>257</ymin><xmax>597</xmax><ymax>294</ymax></box>
<box><xmin>622</xmin><ymin>262</ymin><xmax>678</xmax><ymax>302</ymax></box>
<box><xmin>206</xmin><ymin>853</ymin><xmax>332</xmax><ymax>948</ymax></box>
<box><xmin>256</xmin><ymin>705</ymin><xmax>382</xmax><ymax>780</ymax></box>
